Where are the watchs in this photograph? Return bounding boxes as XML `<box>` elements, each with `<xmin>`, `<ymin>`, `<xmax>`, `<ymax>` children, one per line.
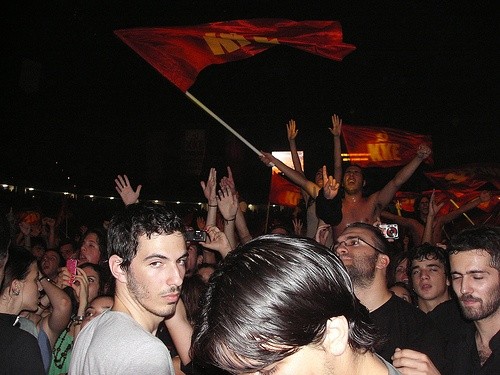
<box><xmin>37</xmin><ymin>302</ymin><xmax>47</xmax><ymax>310</ymax></box>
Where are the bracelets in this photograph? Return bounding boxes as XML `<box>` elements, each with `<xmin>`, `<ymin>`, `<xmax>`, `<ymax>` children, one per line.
<box><xmin>223</xmin><ymin>217</ymin><xmax>236</xmax><ymax>223</ymax></box>
<box><xmin>75</xmin><ymin>316</ymin><xmax>86</xmax><ymax>321</ymax></box>
<box><xmin>74</xmin><ymin>321</ymin><xmax>82</xmax><ymax>327</ymax></box>
<box><xmin>208</xmin><ymin>204</ymin><xmax>217</xmax><ymax>207</ymax></box>
<box><xmin>39</xmin><ymin>275</ymin><xmax>56</xmax><ymax>284</ymax></box>
<box><xmin>24</xmin><ymin>232</ymin><xmax>30</xmax><ymax>237</ymax></box>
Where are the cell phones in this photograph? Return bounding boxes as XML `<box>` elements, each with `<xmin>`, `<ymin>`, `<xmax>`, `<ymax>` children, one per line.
<box><xmin>66</xmin><ymin>258</ymin><xmax>76</xmax><ymax>284</ymax></box>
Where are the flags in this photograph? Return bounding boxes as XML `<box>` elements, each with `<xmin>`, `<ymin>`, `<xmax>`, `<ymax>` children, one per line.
<box><xmin>391</xmin><ymin>190</ymin><xmax>457</xmax><ymax>223</ymax></box>
<box><xmin>268</xmin><ymin>170</ymin><xmax>304</xmax><ymax>208</ymax></box>
<box><xmin>425</xmin><ymin>170</ymin><xmax>500</xmax><ymax>211</ymax></box>
<box><xmin>114</xmin><ymin>19</ymin><xmax>356</xmax><ymax>93</ymax></box>
<box><xmin>341</xmin><ymin>124</ymin><xmax>433</xmax><ymax>169</ymax></box>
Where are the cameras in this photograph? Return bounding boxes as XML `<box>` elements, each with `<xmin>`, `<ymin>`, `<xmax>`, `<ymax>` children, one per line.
<box><xmin>378</xmin><ymin>224</ymin><xmax>398</xmax><ymax>239</ymax></box>
<box><xmin>184</xmin><ymin>230</ymin><xmax>206</xmax><ymax>242</ymax></box>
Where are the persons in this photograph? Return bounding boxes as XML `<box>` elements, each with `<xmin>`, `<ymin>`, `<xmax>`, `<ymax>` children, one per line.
<box><xmin>0</xmin><ymin>114</ymin><xmax>500</xmax><ymax>375</ymax></box>
<box><xmin>68</xmin><ymin>202</ymin><xmax>188</xmax><ymax>375</ymax></box>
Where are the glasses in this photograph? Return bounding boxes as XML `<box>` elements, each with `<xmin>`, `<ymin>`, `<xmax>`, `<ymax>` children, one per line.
<box><xmin>419</xmin><ymin>201</ymin><xmax>430</xmax><ymax>207</ymax></box>
<box><xmin>329</xmin><ymin>237</ymin><xmax>385</xmax><ymax>256</ymax></box>
